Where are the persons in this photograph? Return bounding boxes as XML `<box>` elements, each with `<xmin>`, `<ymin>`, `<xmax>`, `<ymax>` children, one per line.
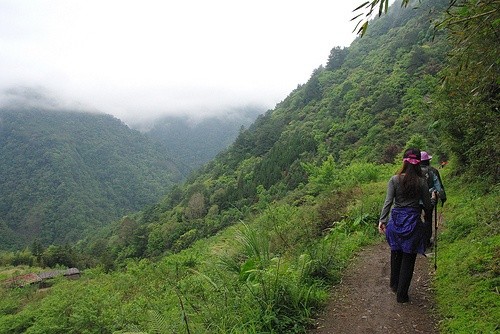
<box><xmin>378</xmin><ymin>146</ymin><xmax>437</xmax><ymax>303</ymax></box>
<box><xmin>417</xmin><ymin>150</ymin><xmax>448</xmax><ymax>248</ymax></box>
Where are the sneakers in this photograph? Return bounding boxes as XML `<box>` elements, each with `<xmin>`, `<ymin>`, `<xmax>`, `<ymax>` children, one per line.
<box><xmin>397</xmin><ymin>294</ymin><xmax>409</xmax><ymax>303</ymax></box>
<box><xmin>390</xmin><ymin>284</ymin><xmax>397</xmax><ymax>293</ymax></box>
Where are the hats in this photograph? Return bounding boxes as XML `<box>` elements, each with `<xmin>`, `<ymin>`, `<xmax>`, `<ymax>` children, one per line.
<box><xmin>420</xmin><ymin>151</ymin><xmax>432</xmax><ymax>160</ymax></box>
<box><xmin>403</xmin><ymin>147</ymin><xmax>420</xmax><ymax>164</ymax></box>
